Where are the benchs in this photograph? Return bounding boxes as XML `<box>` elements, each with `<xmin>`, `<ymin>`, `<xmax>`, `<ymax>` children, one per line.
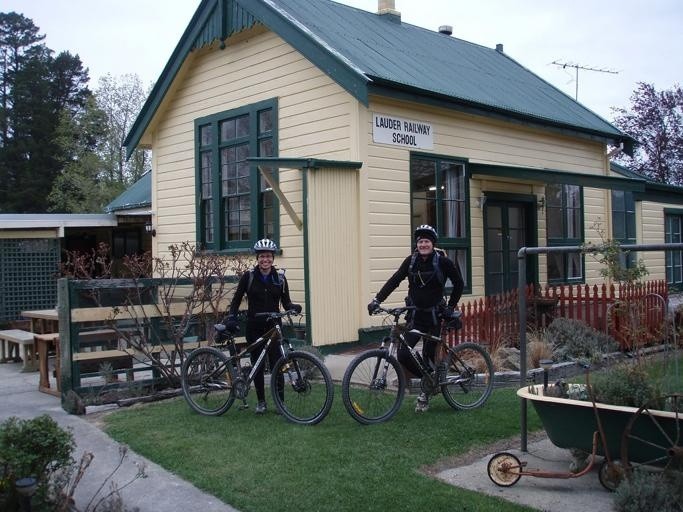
<box><xmin>0</xmin><ymin>296</ymin><xmax>256</xmax><ymax>399</ymax></box>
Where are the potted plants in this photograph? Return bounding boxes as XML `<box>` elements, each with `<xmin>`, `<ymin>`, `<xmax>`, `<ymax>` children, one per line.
<box><xmin>516</xmin><ymin>361</ymin><xmax>682</xmax><ymax>470</ymax></box>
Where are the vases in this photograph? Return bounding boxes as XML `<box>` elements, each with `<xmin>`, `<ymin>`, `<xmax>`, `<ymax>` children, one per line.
<box><xmin>282</xmin><ymin>325</ymin><xmax>296</xmax><ymax>338</ymax></box>
<box><xmin>293</xmin><ymin>327</ymin><xmax>306</xmax><ymax>340</ymax></box>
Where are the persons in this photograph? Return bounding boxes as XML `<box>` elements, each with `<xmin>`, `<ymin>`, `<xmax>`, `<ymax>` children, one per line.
<box><xmin>227</xmin><ymin>238</ymin><xmax>302</xmax><ymax>415</ymax></box>
<box><xmin>367</xmin><ymin>225</ymin><xmax>464</xmax><ymax>412</ymax></box>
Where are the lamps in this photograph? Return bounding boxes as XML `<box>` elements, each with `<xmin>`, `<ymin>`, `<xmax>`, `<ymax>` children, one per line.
<box><xmin>537</xmin><ymin>193</ymin><xmax>545</xmax><ymax>215</ymax></box>
<box><xmin>145</xmin><ymin>215</ymin><xmax>155</xmax><ymax>238</ymax></box>
<box><xmin>474</xmin><ymin>192</ymin><xmax>487</xmax><ymax>217</ymax></box>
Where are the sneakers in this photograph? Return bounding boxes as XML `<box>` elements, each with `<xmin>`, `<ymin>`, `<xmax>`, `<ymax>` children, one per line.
<box><xmin>255</xmin><ymin>401</ymin><xmax>267</xmax><ymax>414</ymax></box>
<box><xmin>414</xmin><ymin>390</ymin><xmax>430</xmax><ymax>413</ymax></box>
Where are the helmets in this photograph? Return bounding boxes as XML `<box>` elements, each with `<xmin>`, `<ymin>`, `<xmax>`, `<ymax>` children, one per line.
<box><xmin>253</xmin><ymin>238</ymin><xmax>278</xmax><ymax>253</ymax></box>
<box><xmin>415</xmin><ymin>224</ymin><xmax>438</xmax><ymax>241</ymax></box>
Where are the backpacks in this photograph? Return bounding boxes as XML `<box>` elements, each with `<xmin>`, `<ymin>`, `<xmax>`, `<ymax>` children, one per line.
<box><xmin>409</xmin><ymin>246</ymin><xmax>448</xmax><ymax>291</ymax></box>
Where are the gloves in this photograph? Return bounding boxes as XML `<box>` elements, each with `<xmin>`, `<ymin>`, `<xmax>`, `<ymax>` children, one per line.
<box><xmin>228</xmin><ymin>313</ymin><xmax>241</xmax><ymax>321</ymax></box>
<box><xmin>290</xmin><ymin>303</ymin><xmax>302</xmax><ymax>316</ymax></box>
<box><xmin>368</xmin><ymin>301</ymin><xmax>380</xmax><ymax>316</ymax></box>
<box><xmin>441</xmin><ymin>305</ymin><xmax>453</xmax><ymax>323</ymax></box>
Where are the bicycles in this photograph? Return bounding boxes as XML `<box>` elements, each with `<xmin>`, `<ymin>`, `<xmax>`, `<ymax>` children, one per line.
<box><xmin>342</xmin><ymin>306</ymin><xmax>494</xmax><ymax>425</ymax></box>
<box><xmin>182</xmin><ymin>308</ymin><xmax>333</xmax><ymax>425</ymax></box>
